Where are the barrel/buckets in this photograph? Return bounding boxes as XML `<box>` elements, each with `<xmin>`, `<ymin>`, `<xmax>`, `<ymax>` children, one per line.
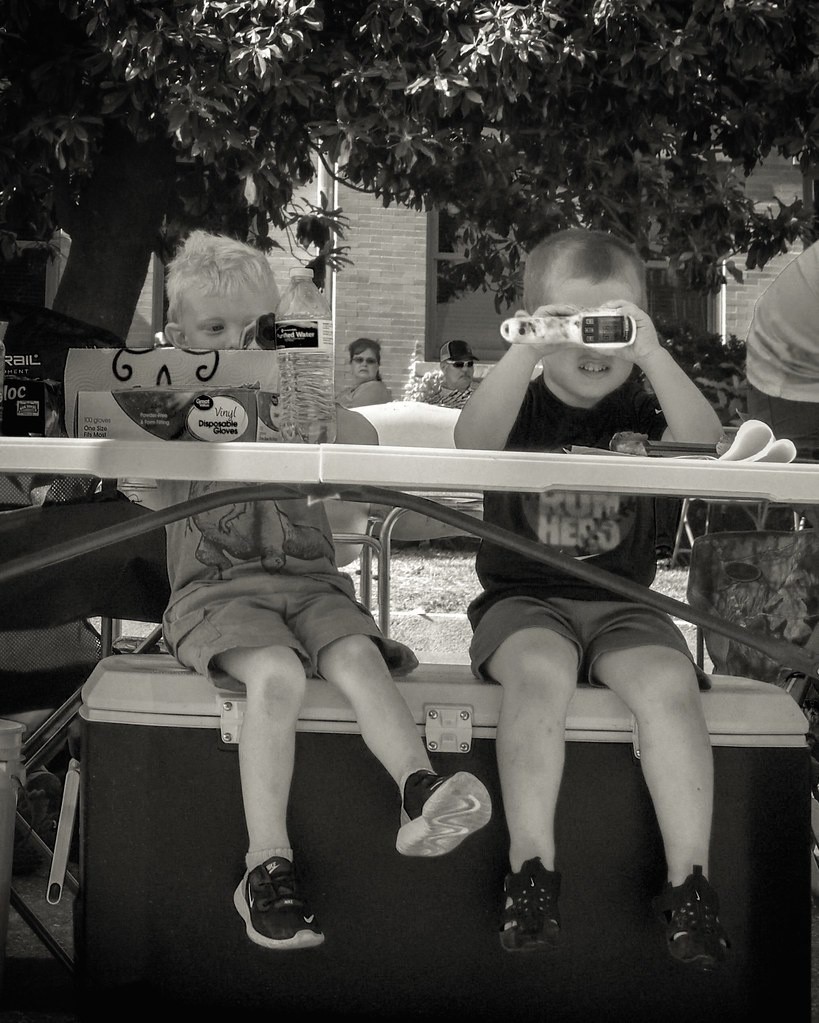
<box><xmin>0</xmin><ymin>719</ymin><xmax>27</xmax><ymax>956</ymax></box>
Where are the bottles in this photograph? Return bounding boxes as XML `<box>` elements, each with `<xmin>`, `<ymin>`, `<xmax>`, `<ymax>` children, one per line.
<box><xmin>274</xmin><ymin>268</ymin><xmax>337</xmax><ymax>444</ymax></box>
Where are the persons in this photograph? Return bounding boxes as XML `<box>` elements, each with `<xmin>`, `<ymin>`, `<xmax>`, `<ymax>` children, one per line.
<box><xmin>453</xmin><ymin>231</ymin><xmax>730</xmax><ymax>971</ymax></box>
<box><xmin>747</xmin><ymin>240</ymin><xmax>819</xmax><ymax>800</ymax></box>
<box><xmin>419</xmin><ymin>339</ymin><xmax>479</xmax><ymax>408</ymax></box>
<box><xmin>333</xmin><ymin>338</ymin><xmax>392</xmax><ymax>407</ymax></box>
<box><xmin>117</xmin><ymin>229</ymin><xmax>491</xmax><ymax>948</ymax></box>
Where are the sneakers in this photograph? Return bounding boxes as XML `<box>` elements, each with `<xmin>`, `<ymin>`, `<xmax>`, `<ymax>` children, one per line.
<box><xmin>234</xmin><ymin>857</ymin><xmax>325</xmax><ymax>950</ymax></box>
<box><xmin>396</xmin><ymin>762</ymin><xmax>493</xmax><ymax>856</ymax></box>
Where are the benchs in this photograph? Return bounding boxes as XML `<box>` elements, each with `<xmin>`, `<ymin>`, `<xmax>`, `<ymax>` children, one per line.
<box><xmin>347</xmin><ymin>398</ymin><xmax>485</xmax><ymax>542</ymax></box>
<box><xmin>69</xmin><ymin>655</ymin><xmax>814</xmax><ymax>1023</ymax></box>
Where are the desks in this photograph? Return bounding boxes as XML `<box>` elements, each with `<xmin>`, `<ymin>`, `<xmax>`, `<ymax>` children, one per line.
<box><xmin>2</xmin><ymin>433</ymin><xmax>819</xmax><ymax>688</ymax></box>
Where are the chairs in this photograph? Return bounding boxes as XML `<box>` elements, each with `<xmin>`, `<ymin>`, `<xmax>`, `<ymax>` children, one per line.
<box><xmin>309</xmin><ymin>404</ymin><xmax>798</xmax><ymax>639</ymax></box>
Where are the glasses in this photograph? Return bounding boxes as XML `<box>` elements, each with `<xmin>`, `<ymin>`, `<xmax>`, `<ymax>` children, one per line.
<box><xmin>350</xmin><ymin>356</ymin><xmax>378</xmax><ymax>365</ymax></box>
<box><xmin>446</xmin><ymin>359</ymin><xmax>474</xmax><ymax>369</ymax></box>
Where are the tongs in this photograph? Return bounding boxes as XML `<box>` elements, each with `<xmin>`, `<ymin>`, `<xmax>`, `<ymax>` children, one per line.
<box><xmin>610</xmin><ymin>431</ymin><xmax>717</xmax><ymax>456</ymax></box>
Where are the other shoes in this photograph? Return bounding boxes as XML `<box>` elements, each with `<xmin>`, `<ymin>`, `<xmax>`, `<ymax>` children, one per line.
<box><xmin>655</xmin><ymin>545</ymin><xmax>674</xmax><ymax>565</ymax></box>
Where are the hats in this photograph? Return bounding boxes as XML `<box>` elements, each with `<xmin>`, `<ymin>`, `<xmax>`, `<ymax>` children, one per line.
<box><xmin>440</xmin><ymin>340</ymin><xmax>480</xmax><ymax>363</ymax></box>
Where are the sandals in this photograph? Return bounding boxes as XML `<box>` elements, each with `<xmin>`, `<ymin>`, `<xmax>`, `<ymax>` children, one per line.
<box><xmin>500</xmin><ymin>856</ymin><xmax>564</xmax><ymax>955</ymax></box>
<box><xmin>657</xmin><ymin>864</ymin><xmax>731</xmax><ymax>972</ymax></box>
